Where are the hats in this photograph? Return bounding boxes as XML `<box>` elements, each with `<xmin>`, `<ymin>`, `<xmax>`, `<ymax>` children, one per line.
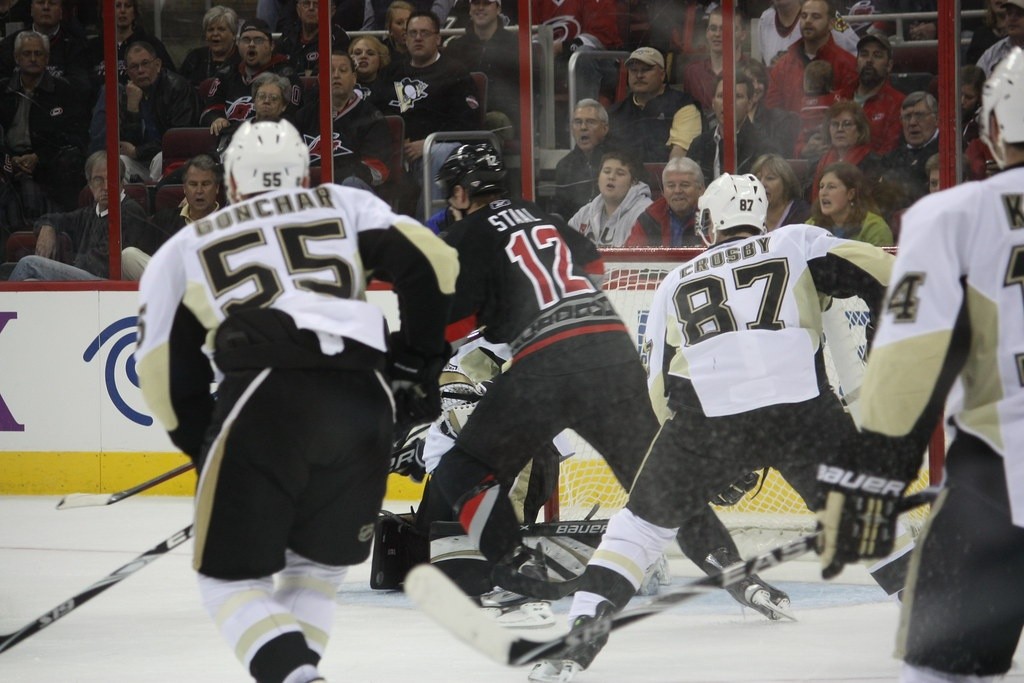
<box><xmin>470</xmin><ymin>0</ymin><xmax>501</xmax><ymax>8</ymax></box>
<box><xmin>624</xmin><ymin>47</ymin><xmax>666</xmax><ymax>71</ymax></box>
<box><xmin>241</xmin><ymin>18</ymin><xmax>273</xmax><ymax>37</ymax></box>
<box><xmin>1000</xmin><ymin>0</ymin><xmax>1024</xmax><ymax>11</ymax></box>
<box><xmin>855</xmin><ymin>32</ymin><xmax>893</xmax><ymax>54</ymax></box>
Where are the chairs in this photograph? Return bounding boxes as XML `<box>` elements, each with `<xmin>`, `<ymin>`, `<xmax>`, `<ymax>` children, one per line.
<box><xmin>3</xmin><ymin>72</ymin><xmax>488</xmax><ymax>273</ymax></box>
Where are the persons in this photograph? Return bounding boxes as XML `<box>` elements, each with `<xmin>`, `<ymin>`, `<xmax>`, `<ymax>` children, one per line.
<box><xmin>133</xmin><ymin>118</ymin><xmax>461</xmax><ymax>683</ymax></box>
<box><xmin>548</xmin><ymin>172</ymin><xmax>897</xmax><ymax>671</ymax></box>
<box><xmin>371</xmin><ymin>142</ymin><xmax>792</xmax><ymax>624</ymax></box>
<box><xmin>814</xmin><ymin>45</ymin><xmax>1024</xmax><ymax>682</ymax></box>
<box><xmin>0</xmin><ymin>0</ymin><xmax>1024</xmax><ymax>282</ymax></box>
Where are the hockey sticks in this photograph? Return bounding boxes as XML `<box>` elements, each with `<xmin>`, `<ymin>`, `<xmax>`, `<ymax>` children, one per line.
<box><xmin>0</xmin><ymin>383</ymin><xmax>427</xmax><ymax>653</ymax></box>
<box><xmin>53</xmin><ymin>324</ymin><xmax>488</xmax><ymax>512</ymax></box>
<box><xmin>431</xmin><ymin>519</ymin><xmax>609</xmax><ymax>538</ymax></box>
<box><xmin>390</xmin><ymin>438</ymin><xmax>424</xmax><ymax>473</ymax></box>
<box><xmin>402</xmin><ymin>484</ymin><xmax>941</xmax><ymax>670</ymax></box>
<box><xmin>488</xmin><ymin>562</ymin><xmax>585</xmax><ymax>601</ymax></box>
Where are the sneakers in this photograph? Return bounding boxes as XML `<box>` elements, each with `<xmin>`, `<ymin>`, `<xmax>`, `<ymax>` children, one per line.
<box><xmin>715</xmin><ymin>555</ymin><xmax>801</xmax><ymax>623</ymax></box>
<box><xmin>480</xmin><ymin>542</ymin><xmax>555</xmax><ymax>625</ymax></box>
<box><xmin>635</xmin><ymin>556</ymin><xmax>670</xmax><ymax>597</ymax></box>
<box><xmin>530</xmin><ymin>596</ymin><xmax>616</xmax><ymax>680</ymax></box>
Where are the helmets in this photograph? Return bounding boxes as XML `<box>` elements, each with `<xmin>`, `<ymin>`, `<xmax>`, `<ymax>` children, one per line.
<box><xmin>695</xmin><ymin>171</ymin><xmax>770</xmax><ymax>242</ymax></box>
<box><xmin>439</xmin><ymin>140</ymin><xmax>504</xmax><ymax>198</ymax></box>
<box><xmin>225</xmin><ymin>119</ymin><xmax>313</xmax><ymax>198</ymax></box>
<box><xmin>979</xmin><ymin>46</ymin><xmax>1024</xmax><ymax>149</ymax></box>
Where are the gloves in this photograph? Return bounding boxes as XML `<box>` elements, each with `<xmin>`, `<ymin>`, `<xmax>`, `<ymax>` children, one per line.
<box><xmin>209</xmin><ymin>380</ymin><xmax>396</xmax><ymax>424</ymax></box>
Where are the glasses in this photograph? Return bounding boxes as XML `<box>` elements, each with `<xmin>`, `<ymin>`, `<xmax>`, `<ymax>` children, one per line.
<box><xmin>126</xmin><ymin>58</ymin><xmax>158</xmax><ymax>72</ymax></box>
<box><xmin>830</xmin><ymin>119</ymin><xmax>856</xmax><ymax>130</ymax></box>
<box><xmin>899</xmin><ymin>110</ymin><xmax>934</xmax><ymax>125</ymax></box>
<box><xmin>407</xmin><ymin>30</ymin><xmax>436</xmax><ymax>37</ymax></box>
<box><xmin>256</xmin><ymin>93</ymin><xmax>282</xmax><ymax>102</ymax></box>
<box><xmin>240</xmin><ymin>35</ymin><xmax>268</xmax><ymax>44</ymax></box>
